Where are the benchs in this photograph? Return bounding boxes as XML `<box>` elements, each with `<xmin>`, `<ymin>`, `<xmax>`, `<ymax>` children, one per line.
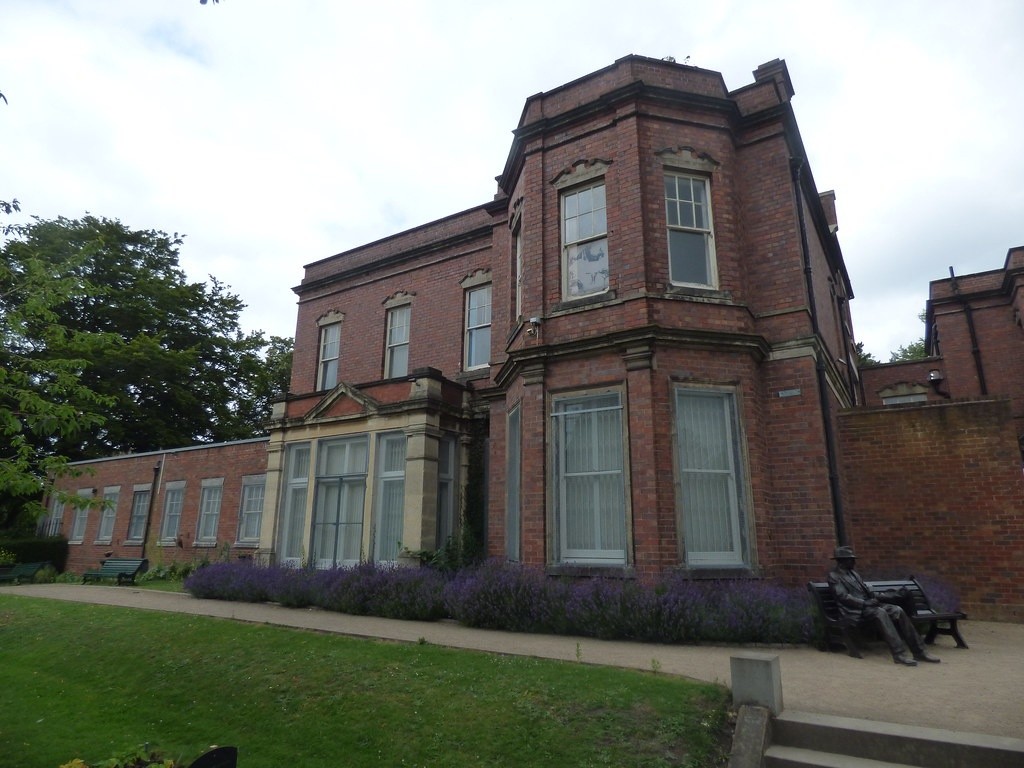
<box><xmin>806</xmin><ymin>575</ymin><xmax>969</xmax><ymax>658</ymax></box>
<box><xmin>81</xmin><ymin>559</ymin><xmax>148</xmax><ymax>586</ymax></box>
<box><xmin>0</xmin><ymin>563</ymin><xmax>42</xmax><ymax>585</ymax></box>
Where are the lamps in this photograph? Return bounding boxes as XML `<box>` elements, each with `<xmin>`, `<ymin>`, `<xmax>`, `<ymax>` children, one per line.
<box><xmin>925</xmin><ymin>368</ymin><xmax>953</xmax><ymax>401</ymax></box>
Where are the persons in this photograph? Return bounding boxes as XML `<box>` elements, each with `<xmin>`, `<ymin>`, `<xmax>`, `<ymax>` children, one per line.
<box><xmin>828</xmin><ymin>546</ymin><xmax>940</xmax><ymax>664</ymax></box>
